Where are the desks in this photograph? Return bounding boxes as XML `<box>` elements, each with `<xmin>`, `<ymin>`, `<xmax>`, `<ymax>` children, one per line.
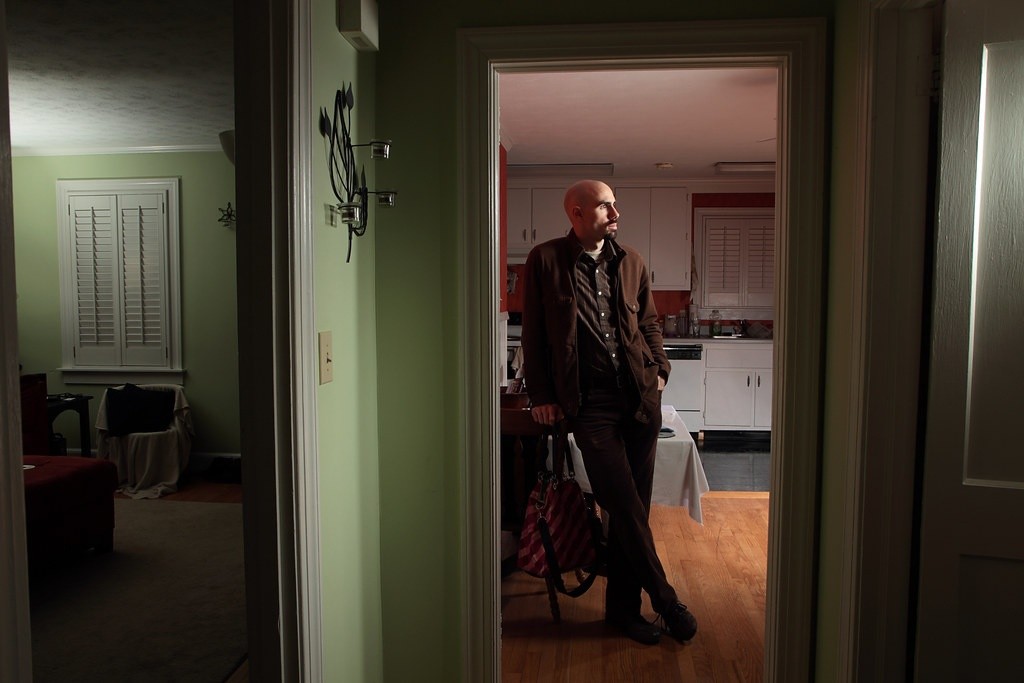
<box><xmin>500</xmin><ymin>394</ymin><xmax>575</xmax><ymax>624</ymax></box>
<box><xmin>544</xmin><ymin>405</ymin><xmax>710</xmax><ymax>540</ymax></box>
<box><xmin>45</xmin><ymin>393</ymin><xmax>93</xmax><ymax>457</ymax></box>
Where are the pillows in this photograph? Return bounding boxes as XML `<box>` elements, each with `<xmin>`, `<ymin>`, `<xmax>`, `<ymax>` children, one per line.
<box><xmin>103</xmin><ymin>384</ymin><xmax>175</xmax><ymax>440</ymax></box>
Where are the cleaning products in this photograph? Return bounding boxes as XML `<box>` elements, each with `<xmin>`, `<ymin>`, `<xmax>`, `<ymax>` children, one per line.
<box><xmin>709</xmin><ymin>310</ymin><xmax>722</xmax><ymax>336</ymax></box>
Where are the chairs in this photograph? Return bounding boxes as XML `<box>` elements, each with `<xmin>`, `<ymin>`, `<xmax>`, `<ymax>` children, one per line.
<box><xmin>94</xmin><ymin>382</ymin><xmax>195</xmax><ymax>500</ymax></box>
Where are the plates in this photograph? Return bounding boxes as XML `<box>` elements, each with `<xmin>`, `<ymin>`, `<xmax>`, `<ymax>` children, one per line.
<box><xmin>23</xmin><ymin>465</ymin><xmax>35</xmax><ymax>470</ymax></box>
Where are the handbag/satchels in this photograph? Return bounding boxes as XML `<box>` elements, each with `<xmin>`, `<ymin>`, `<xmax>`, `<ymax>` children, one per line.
<box><xmin>518</xmin><ymin>417</ymin><xmax>604</xmax><ymax>598</ymax></box>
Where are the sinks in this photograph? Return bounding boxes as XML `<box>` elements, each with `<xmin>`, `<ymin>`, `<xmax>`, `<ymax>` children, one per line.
<box><xmin>713</xmin><ymin>334</ymin><xmax>745</xmax><ymax>339</ymax></box>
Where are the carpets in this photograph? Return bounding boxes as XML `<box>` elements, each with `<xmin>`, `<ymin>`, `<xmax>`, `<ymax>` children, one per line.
<box><xmin>30</xmin><ymin>497</ymin><xmax>249</xmax><ymax>683</ymax></box>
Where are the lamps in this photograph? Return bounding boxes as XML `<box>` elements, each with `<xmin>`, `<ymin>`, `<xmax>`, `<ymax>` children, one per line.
<box><xmin>714</xmin><ymin>162</ymin><xmax>776</xmax><ymax>176</ymax></box>
<box><xmin>506</xmin><ymin>164</ymin><xmax>614</xmax><ymax>179</ymax></box>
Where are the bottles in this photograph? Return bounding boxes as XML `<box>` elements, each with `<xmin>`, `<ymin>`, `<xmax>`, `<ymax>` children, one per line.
<box><xmin>659</xmin><ymin>305</ymin><xmax>722</xmax><ymax>338</ymax></box>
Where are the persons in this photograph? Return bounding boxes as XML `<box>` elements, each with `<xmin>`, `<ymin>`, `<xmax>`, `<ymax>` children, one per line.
<box><xmin>522</xmin><ymin>179</ymin><xmax>695</xmax><ymax>645</ymax></box>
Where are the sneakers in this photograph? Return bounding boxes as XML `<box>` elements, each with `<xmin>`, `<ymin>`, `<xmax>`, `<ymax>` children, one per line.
<box><xmin>605</xmin><ymin>610</ymin><xmax>663</xmax><ymax>645</ymax></box>
<box><xmin>652</xmin><ymin>591</ymin><xmax>697</xmax><ymax>641</ymax></box>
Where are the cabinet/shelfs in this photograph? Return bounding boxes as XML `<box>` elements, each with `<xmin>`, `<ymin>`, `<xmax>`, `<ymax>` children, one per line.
<box><xmin>703</xmin><ymin>371</ymin><xmax>772</xmax><ymax>427</ymax></box>
<box><xmin>506</xmin><ymin>188</ymin><xmax>573</xmax><ymax>244</ymax></box>
<box><xmin>615</xmin><ymin>186</ymin><xmax>688</xmax><ymax>287</ymax></box>
